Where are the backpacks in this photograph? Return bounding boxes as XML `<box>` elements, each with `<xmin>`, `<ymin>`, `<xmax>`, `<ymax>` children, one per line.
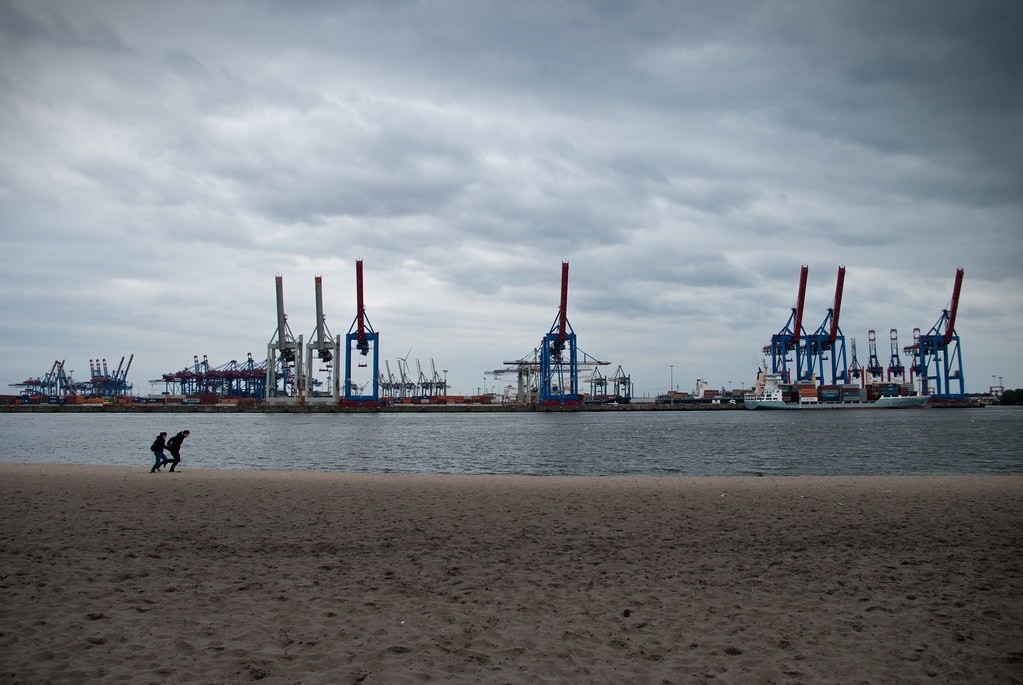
<box><xmin>150</xmin><ymin>442</ymin><xmax>155</xmax><ymax>451</ymax></box>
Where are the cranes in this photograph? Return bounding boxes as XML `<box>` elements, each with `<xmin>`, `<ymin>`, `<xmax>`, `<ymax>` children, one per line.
<box><xmin>761</xmin><ymin>264</ymin><xmax>967</xmax><ymax>402</ymax></box>
<box><xmin>6</xmin><ymin>354</ymin><xmax>139</xmax><ymax>404</ymax></box>
<box><xmin>477</xmin><ymin>258</ymin><xmax>635</xmax><ymax>405</ymax></box>
<box><xmin>150</xmin><ymin>256</ymin><xmax>450</xmax><ymax>402</ymax></box>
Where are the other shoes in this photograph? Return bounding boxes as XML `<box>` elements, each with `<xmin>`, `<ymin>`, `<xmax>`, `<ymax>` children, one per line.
<box><xmin>156</xmin><ymin>468</ymin><xmax>161</xmax><ymax>471</ymax></box>
<box><xmin>151</xmin><ymin>469</ymin><xmax>156</xmax><ymax>473</ymax></box>
<box><xmin>169</xmin><ymin>469</ymin><xmax>174</xmax><ymax>472</ymax></box>
<box><xmin>164</xmin><ymin>463</ymin><xmax>166</xmax><ymax>467</ymax></box>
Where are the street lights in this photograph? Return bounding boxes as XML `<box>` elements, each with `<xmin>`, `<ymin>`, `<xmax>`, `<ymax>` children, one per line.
<box><xmin>670</xmin><ymin>365</ymin><xmax>676</xmax><ymax>405</ymax></box>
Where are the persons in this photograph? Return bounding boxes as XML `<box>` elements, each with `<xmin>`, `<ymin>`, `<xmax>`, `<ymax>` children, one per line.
<box><xmin>149</xmin><ymin>432</ymin><xmax>168</xmax><ymax>472</ymax></box>
<box><xmin>164</xmin><ymin>430</ymin><xmax>190</xmax><ymax>472</ymax></box>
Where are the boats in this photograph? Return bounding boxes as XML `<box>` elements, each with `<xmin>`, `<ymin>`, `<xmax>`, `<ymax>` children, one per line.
<box><xmin>742</xmin><ymin>359</ymin><xmax>984</xmax><ymax>411</ymax></box>
